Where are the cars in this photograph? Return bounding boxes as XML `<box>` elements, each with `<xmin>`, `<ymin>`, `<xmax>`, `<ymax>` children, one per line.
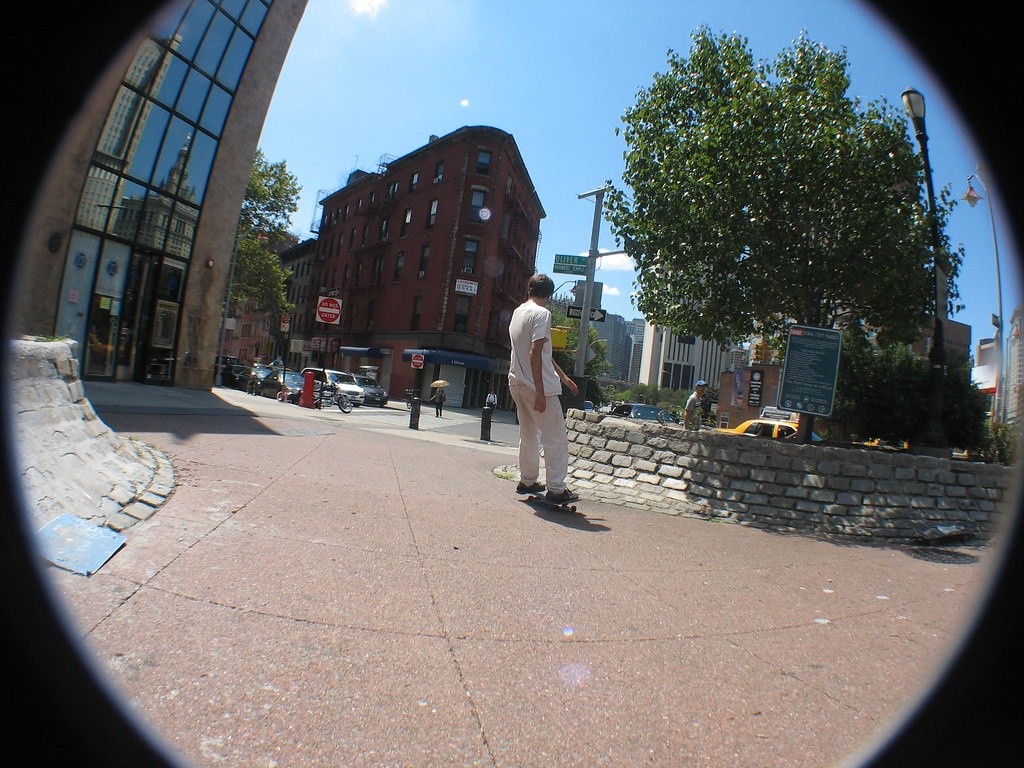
<box><xmin>606</xmin><ymin>402</ymin><xmax>678</xmax><ymax>423</ymax></box>
<box><xmin>951</xmin><ymin>440</ymin><xmax>984</xmax><ymax>462</ymax></box>
<box><xmin>216</xmin><ymin>353</ymin><xmax>291</xmax><ymax>397</ymax></box>
<box><xmin>349</xmin><ymin>372</ymin><xmax>389</xmax><ymax>408</ymax></box>
<box><xmin>257</xmin><ymin>370</ymin><xmax>305</xmax><ymax>404</ymax></box>
<box><xmin>712</xmin><ymin>405</ymin><xmax>826</xmax><ymax>447</ymax></box>
<box><xmin>585</xmin><ymin>401</ymin><xmax>595</xmax><ymax>412</ymax></box>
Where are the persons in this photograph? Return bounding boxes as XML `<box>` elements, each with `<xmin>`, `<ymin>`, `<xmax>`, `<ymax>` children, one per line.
<box><xmin>485</xmin><ymin>390</ymin><xmax>497</xmax><ymax>416</ymax></box>
<box><xmin>507</xmin><ymin>273</ymin><xmax>579</xmax><ymax>504</ymax></box>
<box><xmin>683</xmin><ymin>380</ymin><xmax>709</xmax><ymax>431</ymax></box>
<box><xmin>429</xmin><ymin>387</ymin><xmax>445</xmax><ymax>417</ymax></box>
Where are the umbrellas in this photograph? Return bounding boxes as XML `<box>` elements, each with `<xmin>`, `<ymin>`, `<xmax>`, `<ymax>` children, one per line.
<box><xmin>430</xmin><ymin>379</ymin><xmax>450</xmax><ymax>388</ymax></box>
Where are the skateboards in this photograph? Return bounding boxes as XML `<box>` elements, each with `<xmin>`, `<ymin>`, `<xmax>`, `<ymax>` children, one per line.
<box><xmin>514</xmin><ymin>490</ymin><xmax>580</xmax><ymax>515</ymax></box>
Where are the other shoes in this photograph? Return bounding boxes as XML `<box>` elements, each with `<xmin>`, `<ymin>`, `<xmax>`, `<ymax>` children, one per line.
<box><xmin>545</xmin><ymin>487</ymin><xmax>579</xmax><ymax>503</ymax></box>
<box><xmin>515</xmin><ymin>480</ymin><xmax>545</xmax><ymax>494</ymax></box>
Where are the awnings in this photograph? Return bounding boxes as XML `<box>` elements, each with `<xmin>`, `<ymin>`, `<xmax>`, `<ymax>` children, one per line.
<box><xmin>339</xmin><ymin>346</ymin><xmax>383</xmax><ymax>360</ymax></box>
<box><xmin>401</xmin><ymin>348</ymin><xmax>489</xmax><ymax>370</ymax></box>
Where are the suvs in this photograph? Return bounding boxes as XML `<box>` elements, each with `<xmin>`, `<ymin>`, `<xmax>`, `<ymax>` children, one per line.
<box><xmin>301</xmin><ymin>368</ymin><xmax>365</xmax><ymax>407</ymax></box>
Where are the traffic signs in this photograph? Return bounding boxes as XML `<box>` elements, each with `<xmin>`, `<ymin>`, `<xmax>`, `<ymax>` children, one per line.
<box><xmin>566</xmin><ymin>307</ymin><xmax>608</xmax><ymax>323</ymax></box>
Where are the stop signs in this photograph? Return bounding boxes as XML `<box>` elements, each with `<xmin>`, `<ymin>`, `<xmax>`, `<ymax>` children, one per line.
<box><xmin>412</xmin><ymin>354</ymin><xmax>424</xmax><ymax>368</ymax></box>
<box><xmin>318</xmin><ymin>299</ymin><xmax>340</xmax><ymax>324</ymax></box>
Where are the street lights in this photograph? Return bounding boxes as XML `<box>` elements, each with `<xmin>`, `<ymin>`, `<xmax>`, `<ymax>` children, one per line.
<box><xmin>901</xmin><ymin>84</ymin><xmax>949</xmax><ymax>448</ymax></box>
<box><xmin>960</xmin><ymin>171</ymin><xmax>1004</xmax><ymax>435</ymax></box>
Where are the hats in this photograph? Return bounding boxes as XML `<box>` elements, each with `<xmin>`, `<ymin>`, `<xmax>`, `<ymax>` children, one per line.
<box><xmin>696</xmin><ymin>380</ymin><xmax>708</xmax><ymax>387</ymax></box>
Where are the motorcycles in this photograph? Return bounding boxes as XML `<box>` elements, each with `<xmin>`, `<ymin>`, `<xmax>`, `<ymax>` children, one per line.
<box><xmin>314</xmin><ymin>377</ymin><xmax>354</xmax><ymax>415</ymax></box>
